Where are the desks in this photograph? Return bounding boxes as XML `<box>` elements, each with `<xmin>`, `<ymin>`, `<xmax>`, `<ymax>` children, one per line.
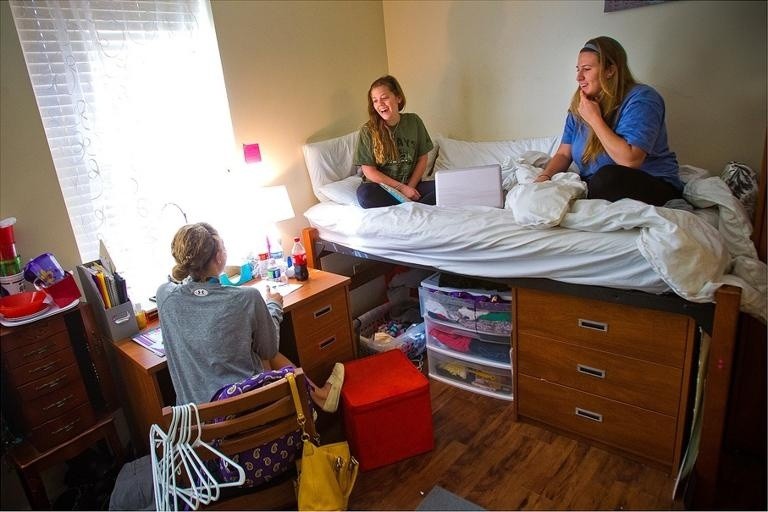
<box><xmin>91</xmin><ymin>264</ymin><xmax>354</xmax><ymax>449</ymax></box>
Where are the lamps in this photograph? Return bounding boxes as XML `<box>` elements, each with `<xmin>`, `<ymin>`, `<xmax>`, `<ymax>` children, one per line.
<box><xmin>246</xmin><ymin>185</ymin><xmax>294</xmax><ymax>225</ymax></box>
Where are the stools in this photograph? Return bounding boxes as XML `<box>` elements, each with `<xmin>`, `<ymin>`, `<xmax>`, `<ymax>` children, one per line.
<box><xmin>4</xmin><ymin>406</ymin><xmax>127</xmax><ymax>511</ymax></box>
<box><xmin>337</xmin><ymin>349</ymin><xmax>435</xmax><ymax>471</ymax></box>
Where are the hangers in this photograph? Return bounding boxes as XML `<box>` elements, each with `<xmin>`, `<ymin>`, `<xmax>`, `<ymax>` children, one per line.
<box><xmin>149</xmin><ymin>404</ymin><xmax>245</xmax><ymax>509</ymax></box>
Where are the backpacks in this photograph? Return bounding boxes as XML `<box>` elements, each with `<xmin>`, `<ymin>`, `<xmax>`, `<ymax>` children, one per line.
<box><xmin>186</xmin><ymin>365</ymin><xmax>317</xmax><ymax>511</ymax></box>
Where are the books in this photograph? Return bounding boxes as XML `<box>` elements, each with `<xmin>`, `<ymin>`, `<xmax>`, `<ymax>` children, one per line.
<box><xmin>85</xmin><ymin>238</ymin><xmax>128</xmax><ymax>309</ymax></box>
<box><xmin>143</xmin><ymin>301</ymin><xmax>158</xmax><ymax>322</ymax></box>
<box><xmin>130</xmin><ymin>325</ymin><xmax>167</xmax><ymax>358</ymax></box>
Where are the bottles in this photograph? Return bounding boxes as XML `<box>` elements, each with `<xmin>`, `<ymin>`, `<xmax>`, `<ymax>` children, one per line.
<box><xmin>271</xmin><ymin>260</ymin><xmax>281</xmax><ymax>284</ymax></box>
<box><xmin>292</xmin><ymin>237</ymin><xmax>309</xmax><ymax>282</ymax></box>
<box><xmin>270</xmin><ymin>237</ymin><xmax>283</xmax><ymax>262</ymax></box>
<box><xmin>258</xmin><ymin>244</ymin><xmax>270</xmax><ymax>280</ymax></box>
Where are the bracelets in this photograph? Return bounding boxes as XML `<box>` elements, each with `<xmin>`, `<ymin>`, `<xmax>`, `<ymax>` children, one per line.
<box><xmin>394</xmin><ymin>183</ymin><xmax>403</xmax><ymax>189</ymax></box>
<box><xmin>539</xmin><ymin>174</ymin><xmax>551</xmax><ymax>180</ymax></box>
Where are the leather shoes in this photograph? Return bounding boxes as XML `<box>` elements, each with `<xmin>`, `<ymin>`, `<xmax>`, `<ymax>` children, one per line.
<box><xmin>321</xmin><ymin>361</ymin><xmax>345</xmax><ymax>414</ymax></box>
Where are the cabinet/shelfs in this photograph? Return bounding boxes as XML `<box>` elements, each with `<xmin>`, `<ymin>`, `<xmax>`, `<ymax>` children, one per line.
<box><xmin>516</xmin><ymin>284</ymin><xmax>696</xmax><ymax>469</ymax></box>
<box><xmin>1</xmin><ymin>305</ymin><xmax>118</xmax><ymax>428</ymax></box>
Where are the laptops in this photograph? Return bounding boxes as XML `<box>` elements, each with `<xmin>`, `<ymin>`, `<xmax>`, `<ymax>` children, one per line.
<box><xmin>436</xmin><ymin>164</ymin><xmax>503</xmax><ymax>208</ymax></box>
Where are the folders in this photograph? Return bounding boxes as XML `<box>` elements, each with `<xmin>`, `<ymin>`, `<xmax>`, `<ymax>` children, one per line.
<box><xmin>76</xmin><ymin>261</ymin><xmax>140</xmax><ymax>343</ymax></box>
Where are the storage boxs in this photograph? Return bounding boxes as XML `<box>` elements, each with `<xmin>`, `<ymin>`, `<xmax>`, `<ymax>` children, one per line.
<box><xmin>425</xmin><ymin>279</ymin><xmax>511</xmax><ymax>399</ymax></box>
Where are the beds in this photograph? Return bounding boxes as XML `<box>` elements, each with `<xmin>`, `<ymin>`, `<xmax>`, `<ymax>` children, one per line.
<box><xmin>304</xmin><ymin>209</ymin><xmax>739</xmax><ymax>511</ymax></box>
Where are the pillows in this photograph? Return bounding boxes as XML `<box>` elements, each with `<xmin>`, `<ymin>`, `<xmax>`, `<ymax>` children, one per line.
<box><xmin>300</xmin><ymin>130</ymin><xmax>366</xmax><ymax>204</ymax></box>
<box><xmin>320</xmin><ymin>174</ymin><xmax>364</xmax><ymax>209</ymax></box>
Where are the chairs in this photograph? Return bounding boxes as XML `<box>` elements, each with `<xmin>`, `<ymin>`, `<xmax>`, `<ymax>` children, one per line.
<box><xmin>158</xmin><ymin>367</ymin><xmax>310</xmax><ymax>511</ymax></box>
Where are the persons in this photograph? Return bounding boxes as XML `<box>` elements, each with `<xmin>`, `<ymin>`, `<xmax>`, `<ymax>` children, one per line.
<box><xmin>352</xmin><ymin>75</ymin><xmax>436</xmax><ymax>209</ymax></box>
<box><xmin>533</xmin><ymin>36</ymin><xmax>684</xmax><ymax>207</ymax></box>
<box><xmin>156</xmin><ymin>222</ymin><xmax>345</xmax><ymax>412</ymax></box>
